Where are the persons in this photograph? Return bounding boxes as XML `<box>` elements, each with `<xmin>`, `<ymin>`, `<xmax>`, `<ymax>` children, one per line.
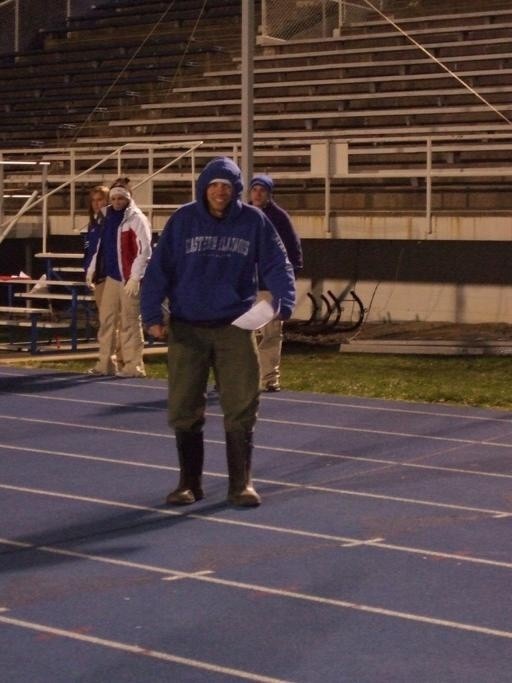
<box><xmin>138</xmin><ymin>154</ymin><xmax>297</xmax><ymax>507</ymax></box>
<box><xmin>205</xmin><ymin>173</ymin><xmax>305</xmax><ymax>398</ymax></box>
<box><xmin>82</xmin><ymin>185</ymin><xmax>126</xmax><ymax>372</ymax></box>
<box><xmin>82</xmin><ymin>177</ymin><xmax>153</xmax><ymax>378</ymax></box>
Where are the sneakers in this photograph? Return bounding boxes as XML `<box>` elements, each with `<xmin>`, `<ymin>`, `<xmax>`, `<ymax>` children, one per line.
<box><xmin>114</xmin><ymin>370</ymin><xmax>147</xmax><ymax>378</ymax></box>
<box><xmin>259</xmin><ymin>378</ymin><xmax>281</xmax><ymax>392</ymax></box>
<box><xmin>88</xmin><ymin>368</ymin><xmax>116</xmax><ymax>376</ymax></box>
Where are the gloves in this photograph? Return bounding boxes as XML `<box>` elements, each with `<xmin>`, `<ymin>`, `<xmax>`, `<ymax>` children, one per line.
<box><xmin>86</xmin><ymin>271</ymin><xmax>97</xmax><ymax>291</ymax></box>
<box><xmin>124</xmin><ymin>279</ymin><xmax>140</xmax><ymax>298</ymax></box>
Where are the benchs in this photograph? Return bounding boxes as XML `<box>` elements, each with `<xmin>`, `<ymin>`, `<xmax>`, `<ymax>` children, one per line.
<box><xmin>1</xmin><ymin>0</ymin><xmax>511</xmax><ymax>213</ymax></box>
<box><xmin>0</xmin><ymin>252</ymin><xmax>100</xmax><ymax>355</ymax></box>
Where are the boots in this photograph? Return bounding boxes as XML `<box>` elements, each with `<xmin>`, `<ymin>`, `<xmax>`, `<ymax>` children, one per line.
<box><xmin>165</xmin><ymin>429</ymin><xmax>205</xmax><ymax>505</ymax></box>
<box><xmin>225</xmin><ymin>430</ymin><xmax>261</xmax><ymax>506</ymax></box>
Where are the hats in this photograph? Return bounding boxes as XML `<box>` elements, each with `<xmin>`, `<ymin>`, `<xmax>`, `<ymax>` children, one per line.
<box><xmin>109</xmin><ymin>178</ymin><xmax>134</xmax><ymax>200</ymax></box>
